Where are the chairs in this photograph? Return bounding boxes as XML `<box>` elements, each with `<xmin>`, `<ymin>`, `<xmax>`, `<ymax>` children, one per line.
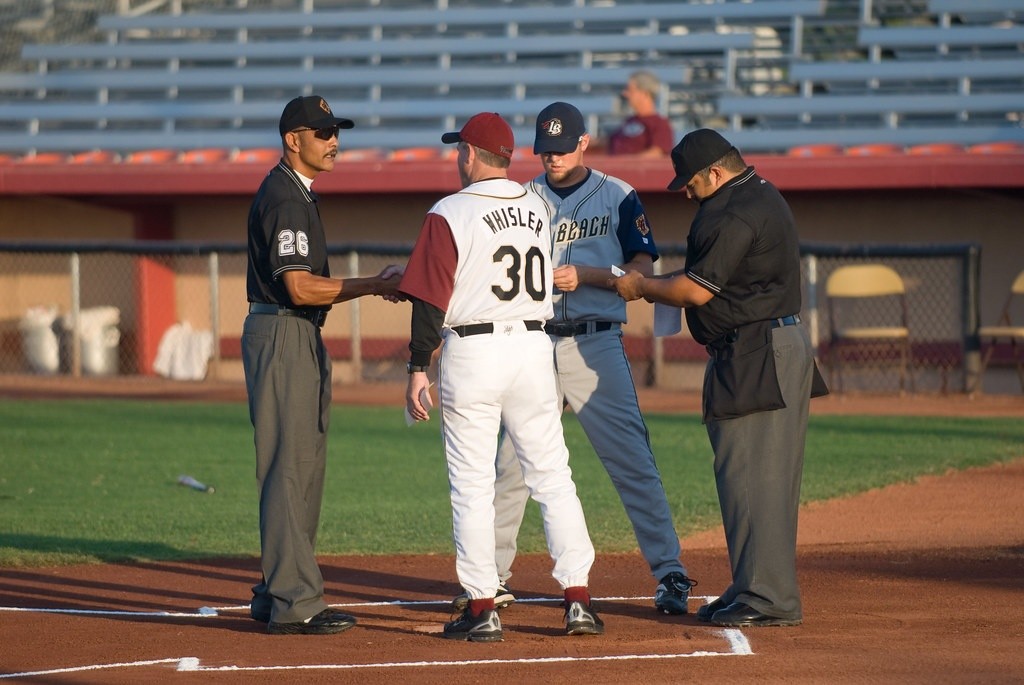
<box><xmin>828</xmin><ymin>264</ymin><xmax>916</xmax><ymax>402</ymax></box>
<box><xmin>967</xmin><ymin>271</ymin><xmax>1024</xmax><ymax>395</ymax></box>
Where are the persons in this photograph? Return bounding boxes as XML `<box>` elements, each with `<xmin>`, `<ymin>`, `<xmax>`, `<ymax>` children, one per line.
<box><xmin>607</xmin><ymin>130</ymin><xmax>829</xmax><ymax>627</ymax></box>
<box><xmin>588</xmin><ymin>73</ymin><xmax>673</xmax><ymax>157</ymax></box>
<box><xmin>380</xmin><ymin>112</ymin><xmax>606</xmax><ymax>642</ymax></box>
<box><xmin>243</xmin><ymin>97</ymin><xmax>409</xmax><ymax>633</ymax></box>
<box><xmin>453</xmin><ymin>101</ymin><xmax>699</xmax><ymax>614</ymax></box>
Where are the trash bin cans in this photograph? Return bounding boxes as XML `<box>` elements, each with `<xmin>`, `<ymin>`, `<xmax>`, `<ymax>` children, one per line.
<box><xmin>16</xmin><ymin>303</ymin><xmax>62</xmax><ymax>373</ymax></box>
<box><xmin>61</xmin><ymin>307</ymin><xmax>122</xmax><ymax>376</ymax></box>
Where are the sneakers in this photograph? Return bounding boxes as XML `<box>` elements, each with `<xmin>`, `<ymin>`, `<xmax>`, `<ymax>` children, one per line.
<box><xmin>250</xmin><ymin>610</ymin><xmax>271</xmax><ymax>623</ymax></box>
<box><xmin>443</xmin><ymin>609</ymin><xmax>503</xmax><ymax>642</ymax></box>
<box><xmin>655</xmin><ymin>573</ymin><xmax>698</xmax><ymax>615</ymax></box>
<box><xmin>452</xmin><ymin>581</ymin><xmax>515</xmax><ymax>612</ymax></box>
<box><xmin>267</xmin><ymin>607</ymin><xmax>357</xmax><ymax>634</ymax></box>
<box><xmin>697</xmin><ymin>598</ymin><xmax>728</xmax><ymax>622</ymax></box>
<box><xmin>561</xmin><ymin>600</ymin><xmax>605</xmax><ymax>635</ymax></box>
<box><xmin>710</xmin><ymin>602</ymin><xmax>803</xmax><ymax>627</ymax></box>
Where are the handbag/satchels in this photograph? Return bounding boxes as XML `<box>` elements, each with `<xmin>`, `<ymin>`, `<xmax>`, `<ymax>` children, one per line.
<box><xmin>811</xmin><ymin>359</ymin><xmax>829</xmax><ymax>398</ymax></box>
<box><xmin>705</xmin><ymin>321</ymin><xmax>787</xmax><ymax>421</ymax></box>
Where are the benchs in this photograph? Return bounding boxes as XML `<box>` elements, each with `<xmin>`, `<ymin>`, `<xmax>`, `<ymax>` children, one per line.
<box><xmin>0</xmin><ymin>0</ymin><xmax>1024</xmax><ymax>151</ymax></box>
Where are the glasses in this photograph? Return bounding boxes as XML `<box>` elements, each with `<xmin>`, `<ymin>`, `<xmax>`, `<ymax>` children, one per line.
<box><xmin>291</xmin><ymin>126</ymin><xmax>339</xmax><ymax>140</ymax></box>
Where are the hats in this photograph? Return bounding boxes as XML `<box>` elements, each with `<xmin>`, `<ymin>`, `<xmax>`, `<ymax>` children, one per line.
<box><xmin>534</xmin><ymin>102</ymin><xmax>585</xmax><ymax>154</ymax></box>
<box><xmin>441</xmin><ymin>112</ymin><xmax>514</xmax><ymax>158</ymax></box>
<box><xmin>667</xmin><ymin>128</ymin><xmax>731</xmax><ymax>191</ymax></box>
<box><xmin>279</xmin><ymin>95</ymin><xmax>354</xmax><ymax>136</ymax></box>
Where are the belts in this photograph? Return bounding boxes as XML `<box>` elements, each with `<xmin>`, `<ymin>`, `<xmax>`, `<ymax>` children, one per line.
<box><xmin>771</xmin><ymin>315</ymin><xmax>801</xmax><ymax>329</ymax></box>
<box><xmin>544</xmin><ymin>320</ymin><xmax>611</xmax><ymax>337</ymax></box>
<box><xmin>451</xmin><ymin>320</ymin><xmax>543</xmax><ymax>337</ymax></box>
<box><xmin>249</xmin><ymin>303</ymin><xmax>327</xmax><ymax>327</ymax></box>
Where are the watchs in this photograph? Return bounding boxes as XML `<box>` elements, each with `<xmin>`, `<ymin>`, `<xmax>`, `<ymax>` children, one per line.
<box><xmin>407</xmin><ymin>362</ymin><xmax>430</xmax><ymax>373</ymax></box>
